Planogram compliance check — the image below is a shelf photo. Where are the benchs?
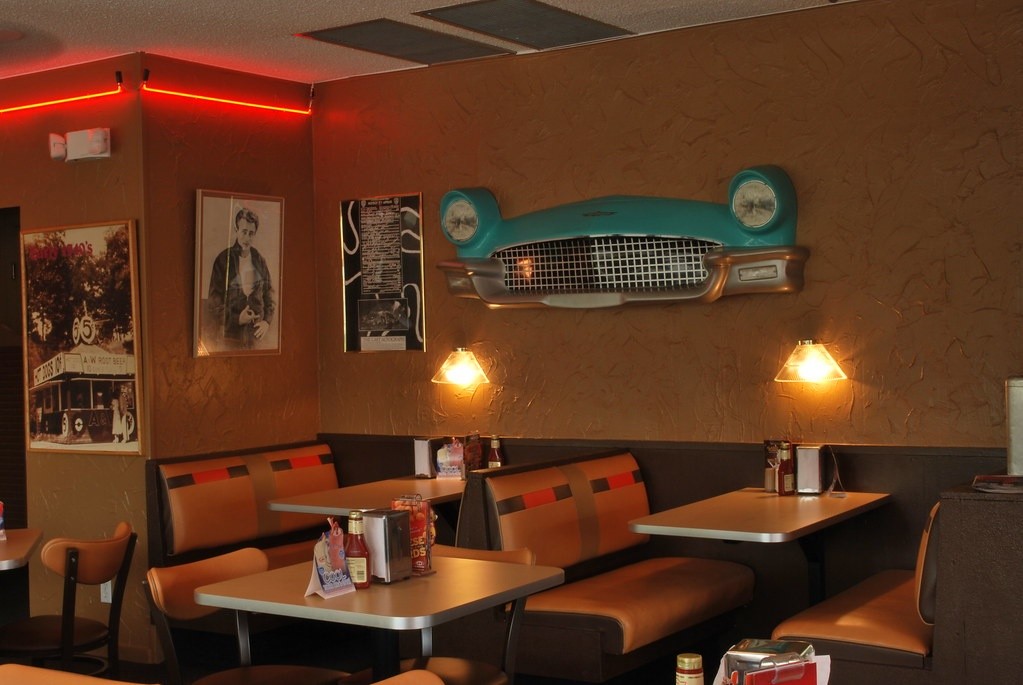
[143,430,947,685]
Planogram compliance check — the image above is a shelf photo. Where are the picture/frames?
[195,189,288,361]
[19,219,145,454]
[339,192,429,355]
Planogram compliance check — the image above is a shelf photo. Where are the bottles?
[675,654,705,685]
[344,511,371,589]
[777,443,795,496]
[488,434,502,468]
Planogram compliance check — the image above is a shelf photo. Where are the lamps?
[771,341,846,384]
[432,349,490,388]
[49,124,112,165]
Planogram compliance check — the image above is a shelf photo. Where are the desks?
[1,527,40,569]
[272,476,468,546]
[628,485,881,621]
[195,555,564,685]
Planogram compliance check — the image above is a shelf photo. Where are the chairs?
[0,520,510,685]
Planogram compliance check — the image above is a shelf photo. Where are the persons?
[209,208,276,350]
[112,388,127,444]
